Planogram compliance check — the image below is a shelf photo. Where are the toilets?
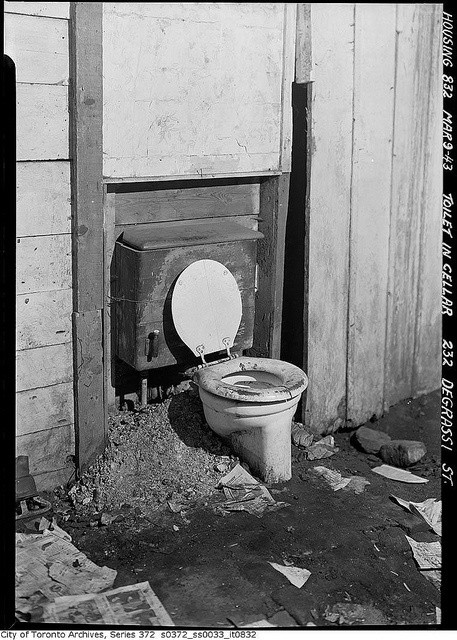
[169,259,307,484]
